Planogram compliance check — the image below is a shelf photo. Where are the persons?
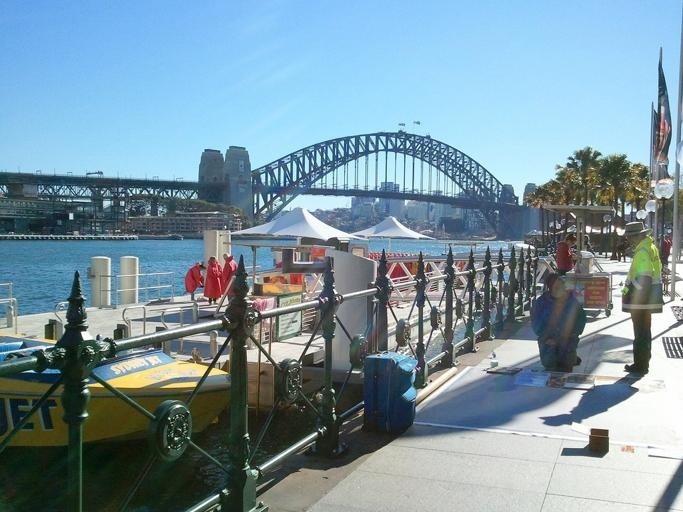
[530,270,587,372]
[556,233,577,276]
[184,262,205,302]
[221,253,238,306]
[204,256,222,306]
[664,234,672,266]
[618,220,664,374]
[657,233,663,249]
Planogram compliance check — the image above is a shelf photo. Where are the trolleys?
[562,272,613,317]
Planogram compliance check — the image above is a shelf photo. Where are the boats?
[1,334,231,447]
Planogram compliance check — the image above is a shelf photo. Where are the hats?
[542,271,558,287]
[622,222,652,238]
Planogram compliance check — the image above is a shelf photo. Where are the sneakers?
[625,364,636,371]
[630,366,649,374]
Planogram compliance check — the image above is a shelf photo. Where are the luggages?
[360,299,418,433]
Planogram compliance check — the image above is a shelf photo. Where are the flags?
[652,61,672,180]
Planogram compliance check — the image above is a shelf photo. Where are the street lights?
[603,213,611,257]
[637,199,657,230]
[653,182,673,258]
